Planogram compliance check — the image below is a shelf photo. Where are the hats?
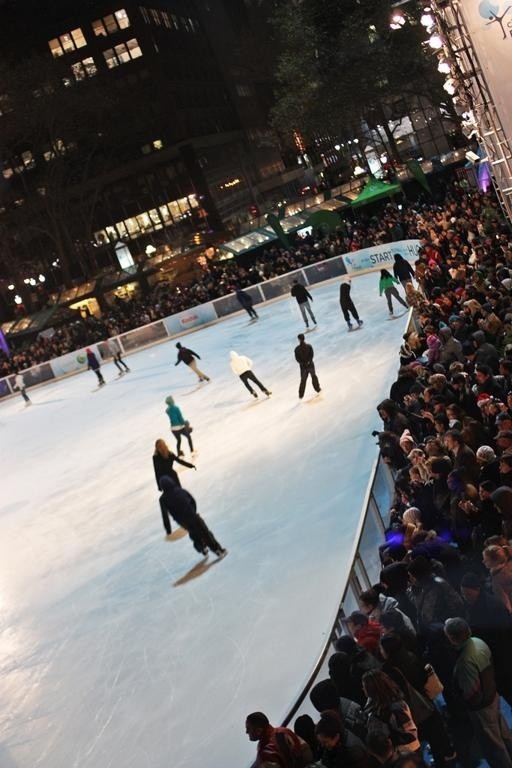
[377,257,512,657]
[341,611,370,629]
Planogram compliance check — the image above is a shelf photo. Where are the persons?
[152,473,230,565]
[244,710,317,767]
[165,394,196,459]
[151,440,197,489]
[236,290,264,320]
[0,274,163,396]
[229,349,273,398]
[173,341,210,382]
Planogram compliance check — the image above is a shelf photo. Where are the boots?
[201,542,228,560]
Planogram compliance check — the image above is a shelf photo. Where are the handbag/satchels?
[388,666,433,722]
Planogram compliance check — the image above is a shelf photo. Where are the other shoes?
[426,742,455,763]
